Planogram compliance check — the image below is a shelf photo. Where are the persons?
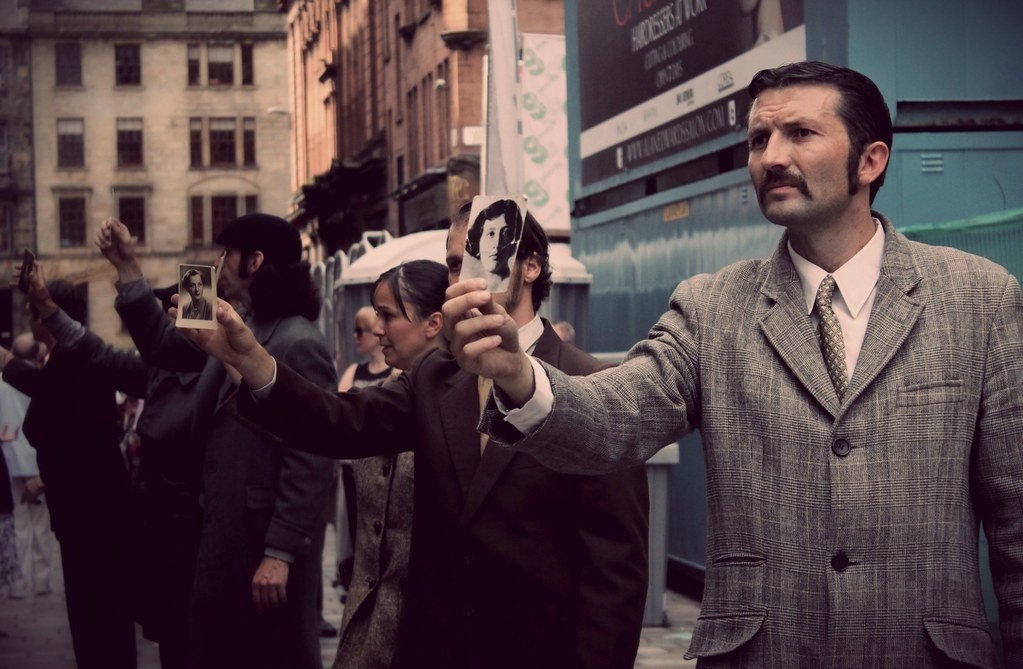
[0,196,650,669]
[181,270,213,321]
[338,306,403,606]
[464,199,524,295]
[439,61,1023,669]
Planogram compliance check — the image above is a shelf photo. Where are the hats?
[10,331,48,363]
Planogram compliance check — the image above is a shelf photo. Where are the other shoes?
[318,617,338,638]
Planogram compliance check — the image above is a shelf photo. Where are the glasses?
[353,325,370,338]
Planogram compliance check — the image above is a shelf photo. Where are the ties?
[813,276,850,404]
[477,376,491,457]
